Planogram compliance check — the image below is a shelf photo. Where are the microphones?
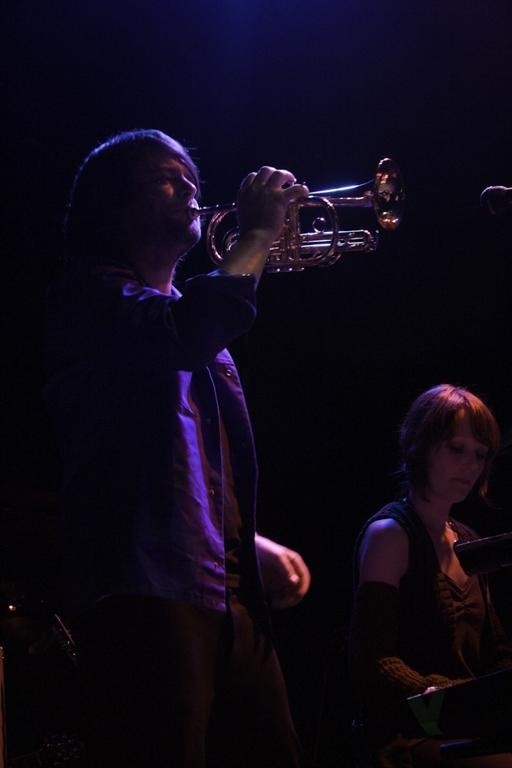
[479,184,512,216]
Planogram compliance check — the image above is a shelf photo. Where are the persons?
[344,383,512,767]
[44,127,313,767]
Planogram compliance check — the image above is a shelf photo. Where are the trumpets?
[194,160,402,276]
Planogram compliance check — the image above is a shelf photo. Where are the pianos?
[407,671,512,744]
